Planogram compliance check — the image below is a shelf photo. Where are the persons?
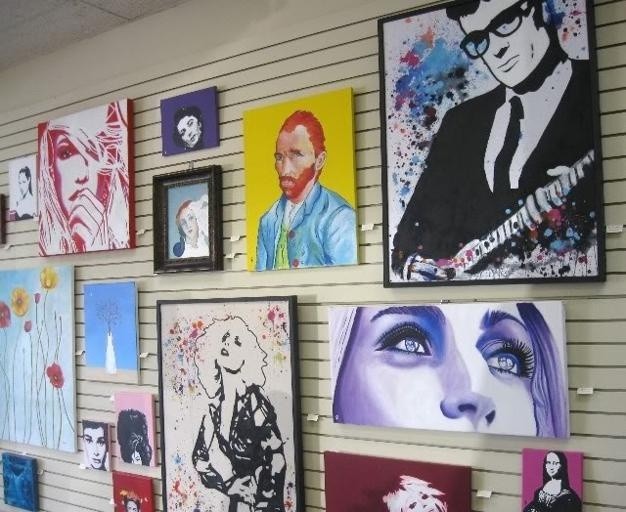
[382,476,448,512]
[14,166,35,220]
[172,106,204,152]
[521,450,582,512]
[327,301,570,439]
[82,419,109,471]
[256,110,358,272]
[116,409,152,467]
[38,99,130,257]
[119,490,142,512]
[191,315,287,512]
[173,201,209,258]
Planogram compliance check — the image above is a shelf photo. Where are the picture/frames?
[152,293,307,512]
[149,163,224,273]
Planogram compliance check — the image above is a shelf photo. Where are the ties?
[274,223,291,270]
[273,149,305,160]
[493,95,524,192]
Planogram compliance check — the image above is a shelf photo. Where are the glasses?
[459,0,536,59]
